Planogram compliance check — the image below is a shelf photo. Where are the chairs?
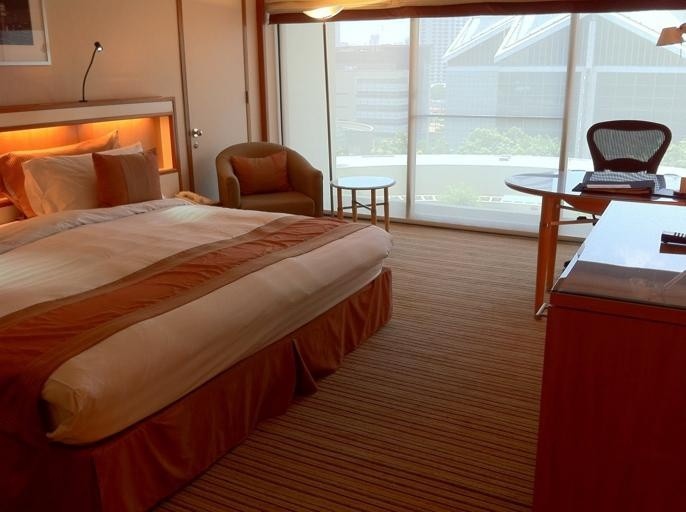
[215,142,323,216]
[585,119,672,174]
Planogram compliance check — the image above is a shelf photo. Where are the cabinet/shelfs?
[530,199,686,512]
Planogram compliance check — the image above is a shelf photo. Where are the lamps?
[79,41,103,102]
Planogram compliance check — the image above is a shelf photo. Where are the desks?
[504,168,686,319]
[330,174,395,232]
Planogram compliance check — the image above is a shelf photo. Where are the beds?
[0,95,392,511]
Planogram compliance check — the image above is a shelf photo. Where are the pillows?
[231,150,291,195]
[20,141,143,217]
[0,129,120,220]
[91,148,164,208]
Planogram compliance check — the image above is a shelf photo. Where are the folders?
[583,171,655,190]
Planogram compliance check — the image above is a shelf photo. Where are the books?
[582,179,655,195]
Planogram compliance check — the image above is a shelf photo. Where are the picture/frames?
[0,0,51,66]
